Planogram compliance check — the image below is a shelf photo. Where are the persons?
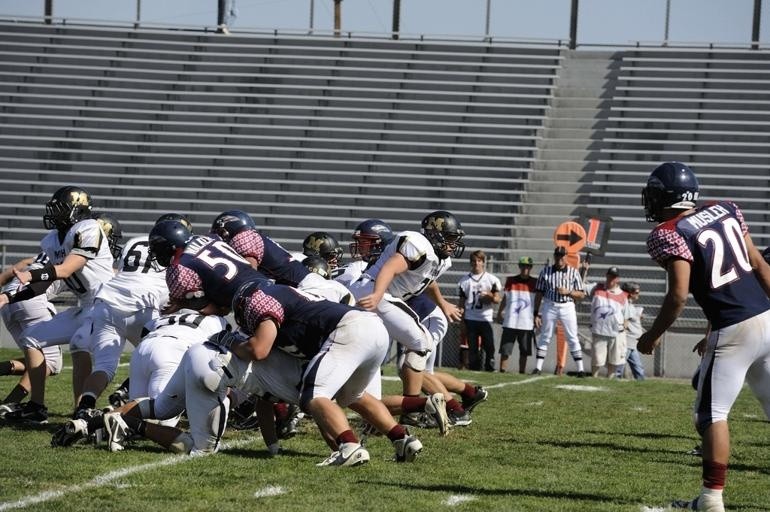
[457,250,501,372]
[613,280,653,382]
[628,159,770,510]
[586,267,628,379]
[2,189,487,467]
[496,255,537,372]
[685,242,769,408]
[530,245,586,379]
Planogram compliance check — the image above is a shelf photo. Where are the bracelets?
[568,289,572,296]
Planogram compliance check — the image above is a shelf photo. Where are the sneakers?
[684,443,702,456]
[0,387,489,468]
[669,486,725,512]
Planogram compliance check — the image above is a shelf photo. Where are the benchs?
[0,21,770,318]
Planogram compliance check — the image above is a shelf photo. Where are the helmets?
[348,219,394,263]
[420,211,465,260]
[301,232,345,265]
[44,187,94,231]
[210,211,256,244]
[96,213,124,261]
[303,255,334,278]
[641,164,700,224]
[154,213,193,235]
[232,280,274,325]
[148,221,194,273]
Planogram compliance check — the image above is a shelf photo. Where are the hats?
[518,256,534,265]
[607,266,618,275]
[553,246,568,257]
